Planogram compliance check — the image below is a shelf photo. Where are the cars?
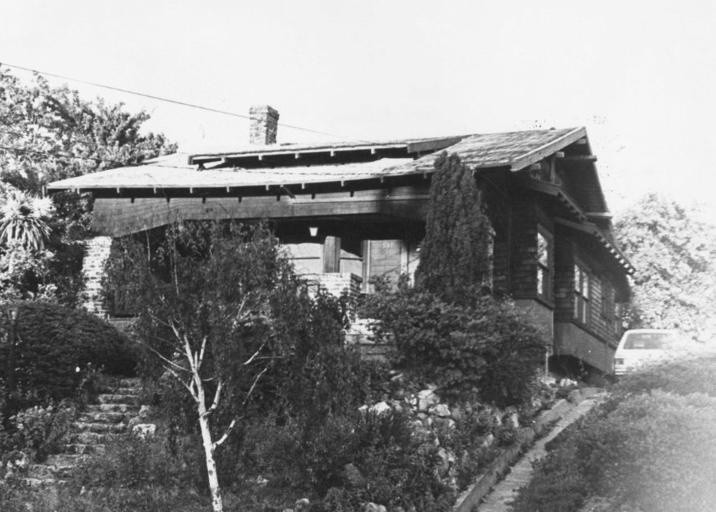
[611,329,686,381]
